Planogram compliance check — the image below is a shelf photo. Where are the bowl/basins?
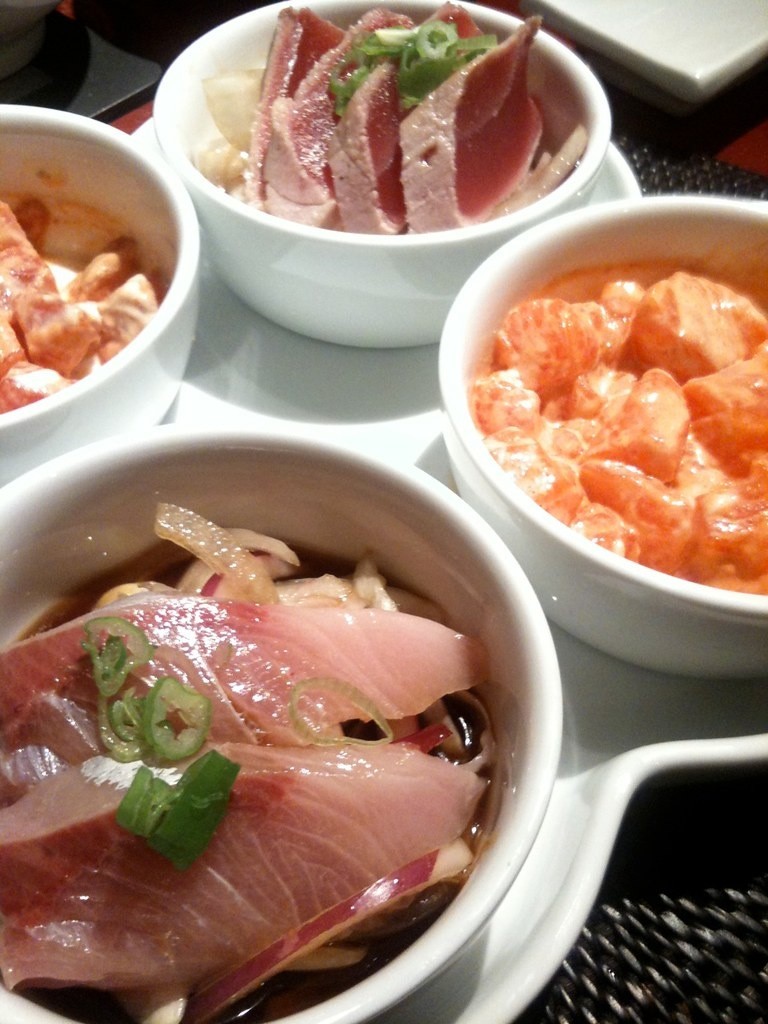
[3,98,205,478]
[442,187,767,685]
[0,1,62,79]
[156,0,612,345]
[0,426,567,1024]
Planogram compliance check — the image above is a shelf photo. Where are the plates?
[5,120,766,1024]
[0,0,252,119]
[520,0,768,114]
